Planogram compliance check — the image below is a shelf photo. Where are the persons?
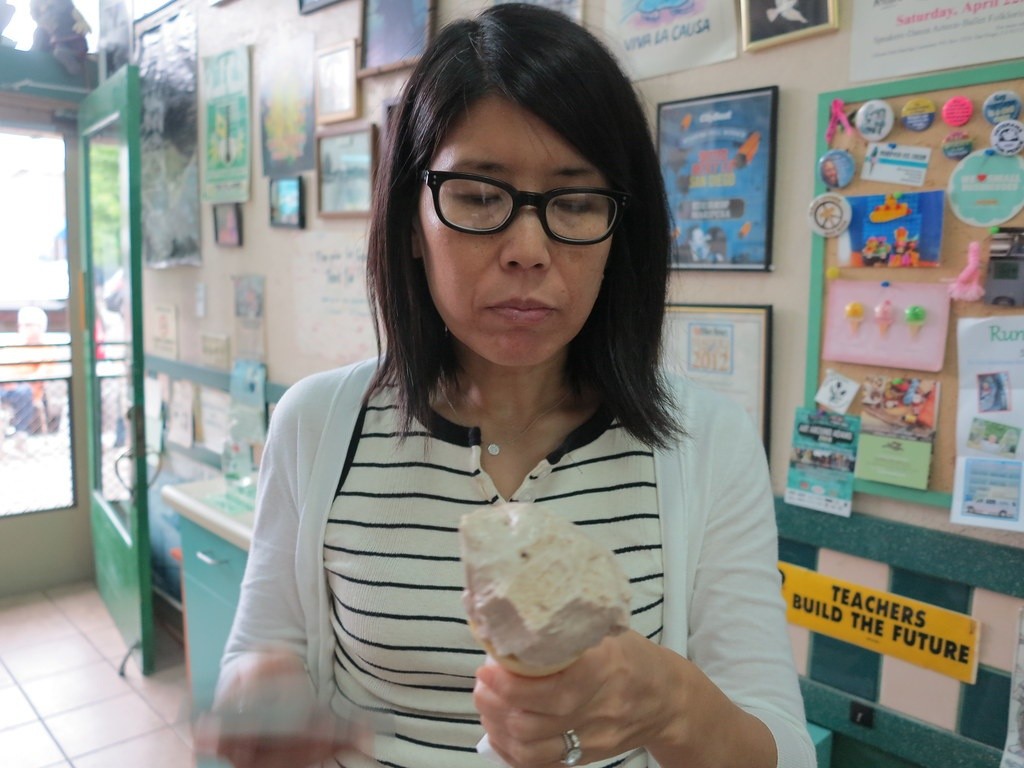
[0,307,56,455]
[193,3,817,768]
[29,0,92,76]
[94,265,132,453]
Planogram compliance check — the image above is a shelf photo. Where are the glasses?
[419,165,626,246]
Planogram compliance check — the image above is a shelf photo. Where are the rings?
[560,729,582,766]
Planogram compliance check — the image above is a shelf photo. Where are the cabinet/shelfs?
[172,518,250,721]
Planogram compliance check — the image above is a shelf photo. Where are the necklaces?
[437,377,573,455]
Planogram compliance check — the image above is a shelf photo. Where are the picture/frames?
[297,0,341,14]
[740,0,839,52]
[213,202,243,246]
[655,86,778,272]
[268,175,306,229]
[315,123,377,218]
[656,301,772,472]
[355,0,436,79]
[314,37,360,124]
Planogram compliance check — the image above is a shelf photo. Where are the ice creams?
[459,502,631,678]
